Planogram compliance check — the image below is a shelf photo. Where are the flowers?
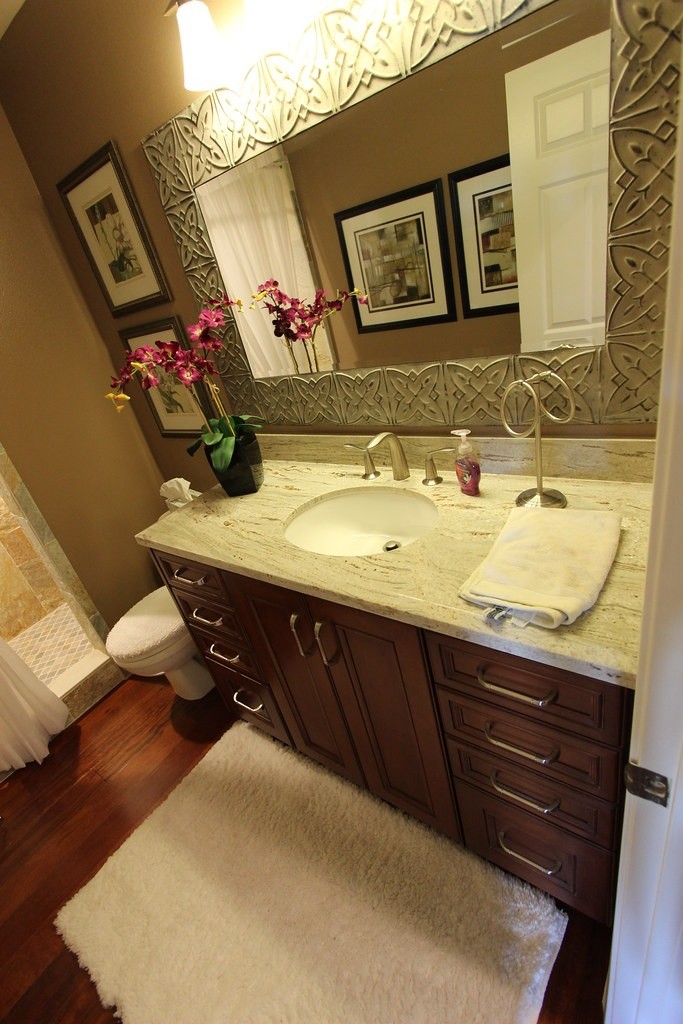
[249,277,369,374]
[104,291,242,413]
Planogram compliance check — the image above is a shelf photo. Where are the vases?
[204,429,264,497]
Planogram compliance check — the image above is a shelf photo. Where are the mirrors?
[140,0,683,427]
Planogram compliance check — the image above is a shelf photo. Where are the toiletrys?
[451,429,480,496]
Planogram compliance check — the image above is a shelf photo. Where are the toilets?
[91,510,216,700]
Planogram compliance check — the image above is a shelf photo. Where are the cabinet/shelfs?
[148,546,635,929]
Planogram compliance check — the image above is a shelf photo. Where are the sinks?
[281,486,439,558]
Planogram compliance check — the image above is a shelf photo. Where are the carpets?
[50,720,568,1024]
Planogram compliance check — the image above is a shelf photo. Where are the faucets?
[366,432,410,481]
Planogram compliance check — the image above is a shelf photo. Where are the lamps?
[162,0,226,92]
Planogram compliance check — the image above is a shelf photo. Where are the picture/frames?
[55,140,174,320]
[117,313,220,439]
[332,177,457,335]
[446,152,521,320]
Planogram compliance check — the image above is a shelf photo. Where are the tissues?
[160,478,203,512]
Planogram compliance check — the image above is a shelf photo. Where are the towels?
[459,508,623,630]
